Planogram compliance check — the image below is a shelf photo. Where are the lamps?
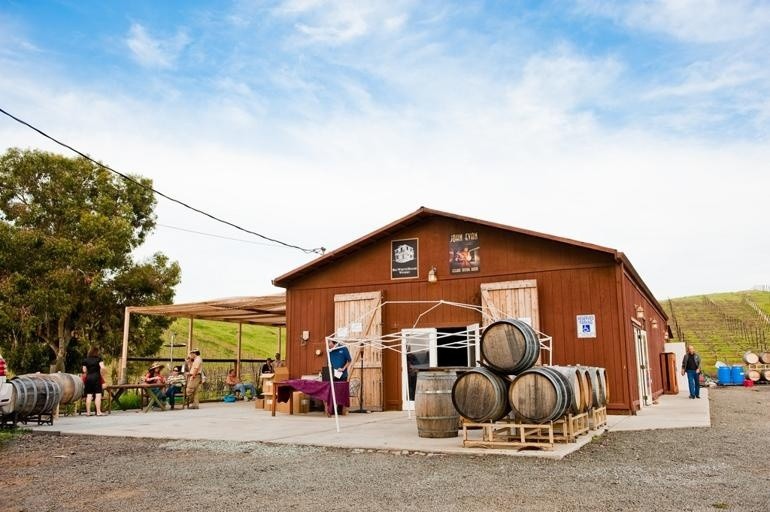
[650,317,657,328]
[634,303,643,318]
[663,330,668,339]
[428,265,437,283]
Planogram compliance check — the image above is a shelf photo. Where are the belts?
[189,373,200,375]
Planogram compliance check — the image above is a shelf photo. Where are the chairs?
[141,377,149,410]
[224,381,247,400]
[79,397,108,414]
[174,376,190,409]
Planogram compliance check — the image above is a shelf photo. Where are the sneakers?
[189,405,199,409]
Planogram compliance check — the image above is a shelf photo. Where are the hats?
[190,348,199,352]
[149,362,163,371]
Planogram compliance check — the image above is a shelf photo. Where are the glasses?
[173,370,179,372]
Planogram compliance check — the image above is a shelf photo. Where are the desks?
[272,381,351,418]
[101,383,166,413]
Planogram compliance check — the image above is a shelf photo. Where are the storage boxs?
[256,367,311,413]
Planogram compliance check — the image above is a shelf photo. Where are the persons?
[326,339,352,416]
[262,357,273,374]
[81,347,108,416]
[144,360,166,411]
[162,364,186,409]
[681,345,701,399]
[184,356,195,374]
[405,344,423,399]
[227,368,257,402]
[270,352,284,373]
[184,347,203,409]
[81,371,107,414]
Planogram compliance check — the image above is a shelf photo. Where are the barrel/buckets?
[452,368,511,421]
[504,367,571,421]
[414,370,458,439]
[2,372,87,418]
[558,364,613,416]
[480,320,540,370]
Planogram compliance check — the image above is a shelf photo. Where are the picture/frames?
[390,238,419,280]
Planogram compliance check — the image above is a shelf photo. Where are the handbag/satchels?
[202,372,206,383]
